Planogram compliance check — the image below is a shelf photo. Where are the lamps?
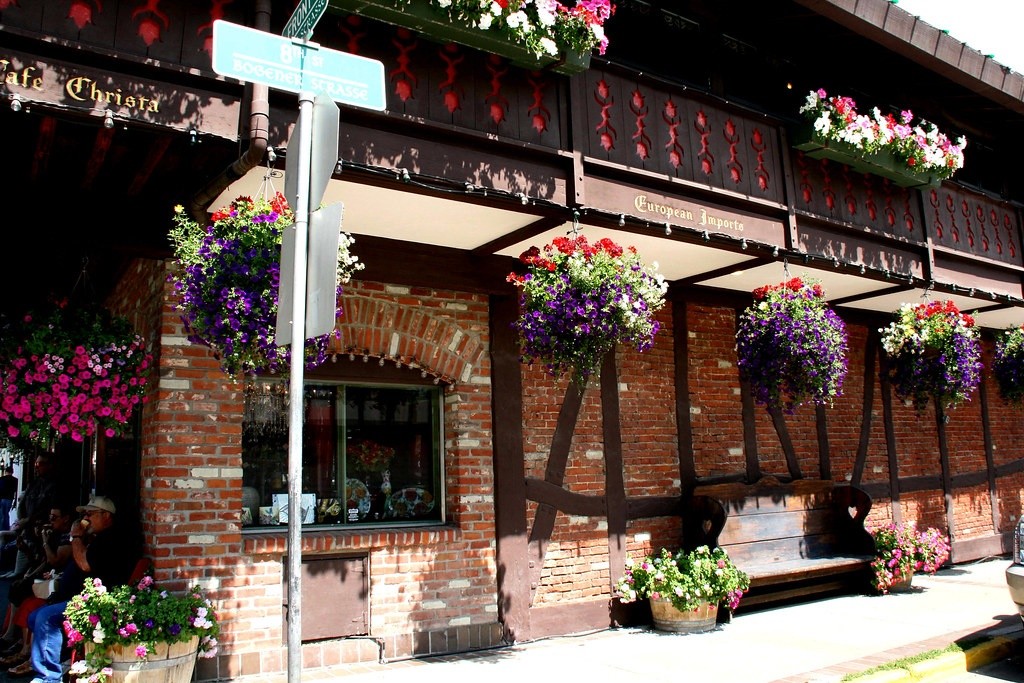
[242,376,307,443]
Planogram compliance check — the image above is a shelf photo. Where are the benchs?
[680,475,878,624]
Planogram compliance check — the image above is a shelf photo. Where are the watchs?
[70,534,82,542]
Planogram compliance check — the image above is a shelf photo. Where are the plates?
[347,478,371,519]
[388,487,435,515]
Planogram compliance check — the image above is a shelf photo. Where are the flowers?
[170,192,365,385]
[800,87,968,182]
[734,273,849,415]
[64,573,221,683]
[878,301,985,423]
[0,297,153,466]
[348,440,395,475]
[391,0,618,60]
[870,520,951,595]
[504,235,669,394]
[612,544,751,613]
[989,323,1024,406]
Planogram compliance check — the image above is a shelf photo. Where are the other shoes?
[0,638,10,648]
[0,653,25,668]
[2,643,23,656]
[6,661,34,678]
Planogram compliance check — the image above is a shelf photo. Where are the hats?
[76,496,116,514]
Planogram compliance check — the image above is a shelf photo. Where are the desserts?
[43,524,52,530]
[81,519,89,528]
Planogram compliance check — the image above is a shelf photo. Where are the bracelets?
[43,542,48,547]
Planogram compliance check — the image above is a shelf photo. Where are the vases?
[85,635,200,683]
[324,0,591,77]
[792,116,942,191]
[363,475,379,510]
[649,592,719,632]
[887,565,914,594]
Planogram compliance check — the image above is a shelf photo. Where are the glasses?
[49,514,61,520]
[82,511,106,517]
[34,462,44,467]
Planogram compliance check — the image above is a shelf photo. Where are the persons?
[0,453,131,676]
[0,467,18,530]
